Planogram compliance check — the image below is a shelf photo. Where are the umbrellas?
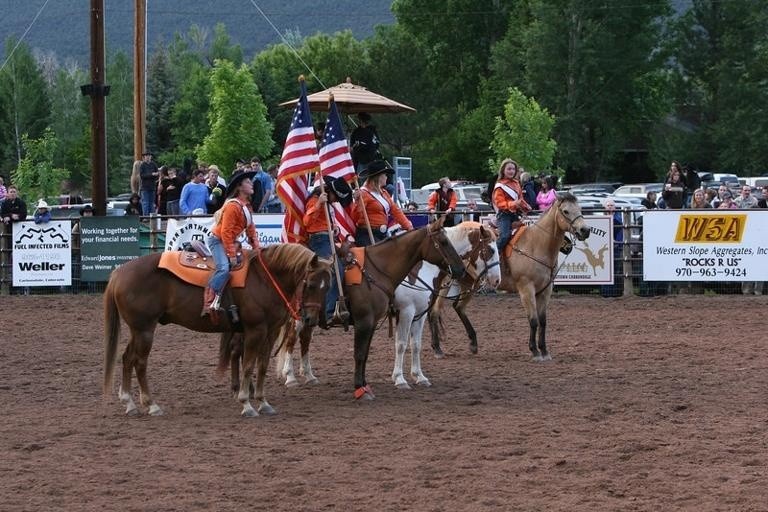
[278,76,419,121]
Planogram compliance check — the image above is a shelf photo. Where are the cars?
[25,192,130,217]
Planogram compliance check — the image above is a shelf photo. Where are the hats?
[358,159,396,180]
[33,199,54,211]
[226,169,257,193]
[487,174,499,199]
[79,205,96,216]
[141,150,155,156]
[322,173,354,208]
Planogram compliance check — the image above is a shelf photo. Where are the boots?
[199,285,226,318]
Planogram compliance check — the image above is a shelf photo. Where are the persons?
[601,161,768,297]
[349,110,380,186]
[201,166,260,325]
[303,176,353,326]
[313,123,325,162]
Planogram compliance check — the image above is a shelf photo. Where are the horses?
[214,213,466,401]
[102,242,340,420]
[275,221,503,390]
[428,188,592,363]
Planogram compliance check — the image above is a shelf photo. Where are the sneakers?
[327,311,351,328]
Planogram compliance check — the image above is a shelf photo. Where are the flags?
[309,92,359,189]
[275,74,322,244]
